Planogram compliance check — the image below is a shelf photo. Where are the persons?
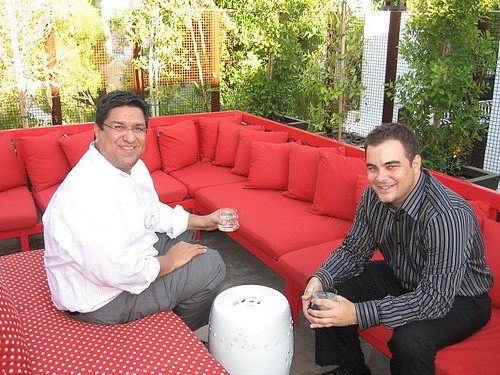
[301,123,495,375]
[41,91,241,332]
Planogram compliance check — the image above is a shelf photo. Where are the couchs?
[0,110,500,375]
[0,244,231,375]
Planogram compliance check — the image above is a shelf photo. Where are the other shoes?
[316,365,372,375]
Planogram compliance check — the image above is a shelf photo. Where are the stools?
[208,284,294,375]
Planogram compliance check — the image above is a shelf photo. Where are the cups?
[217,207,237,232]
[311,285,337,311]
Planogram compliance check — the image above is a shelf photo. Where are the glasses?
[102,123,148,135]
